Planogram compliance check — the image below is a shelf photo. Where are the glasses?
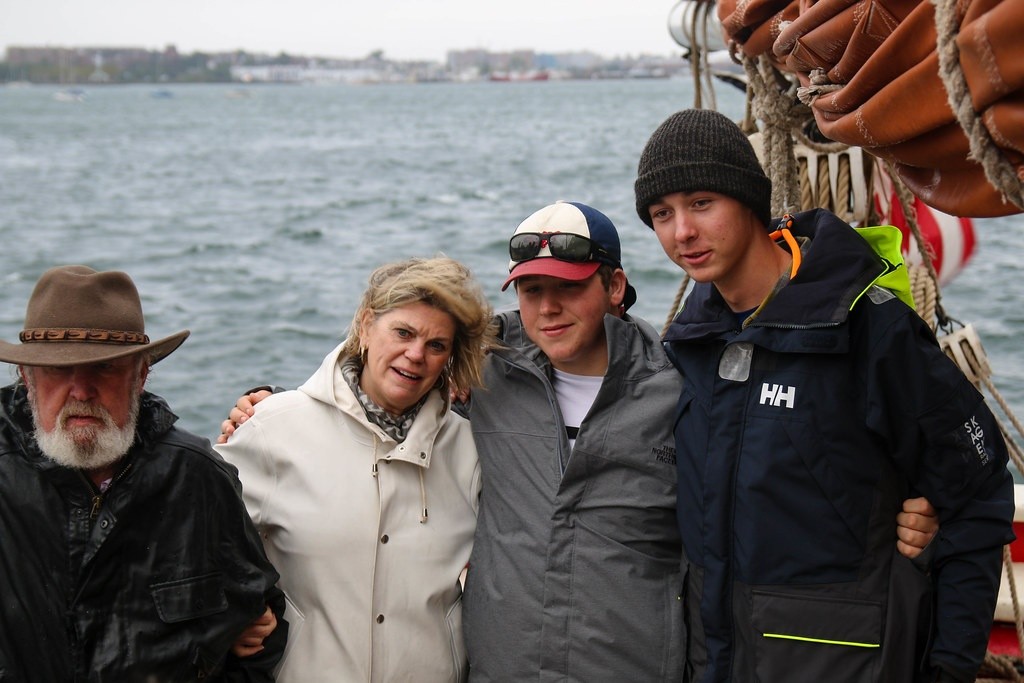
[509,232,621,263]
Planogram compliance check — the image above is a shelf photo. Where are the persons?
[208,255,483,683]
[0,265,286,683]
[220,202,943,683]
[636,109,1015,683]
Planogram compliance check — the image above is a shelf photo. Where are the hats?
[635,109,771,230]
[0,265,191,367]
[501,200,621,292]
